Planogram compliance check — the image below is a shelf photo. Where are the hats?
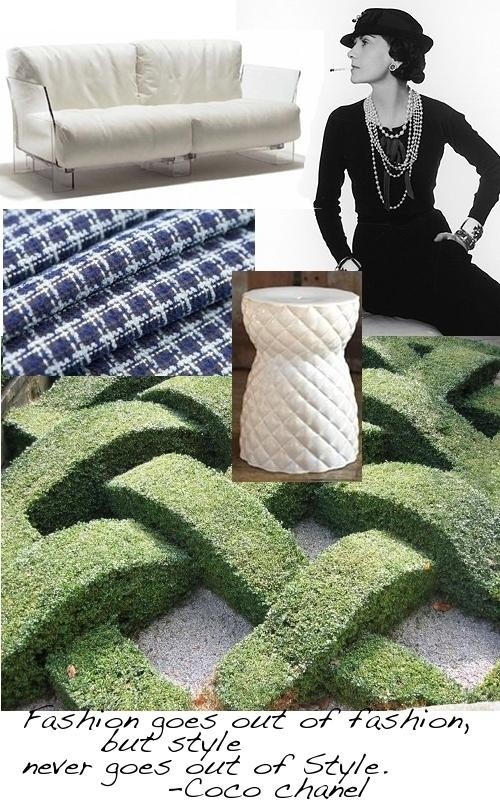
[340,8,433,54]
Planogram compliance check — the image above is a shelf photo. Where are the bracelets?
[456,232,475,250]
[462,215,483,242]
[339,257,363,272]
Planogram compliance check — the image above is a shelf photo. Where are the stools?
[239,286,359,472]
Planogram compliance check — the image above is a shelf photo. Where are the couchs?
[6,40,301,192]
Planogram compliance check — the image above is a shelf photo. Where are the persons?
[312,6,500,336]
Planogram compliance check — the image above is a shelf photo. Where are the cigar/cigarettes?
[330,66,347,72]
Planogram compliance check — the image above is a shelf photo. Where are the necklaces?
[359,87,424,210]
